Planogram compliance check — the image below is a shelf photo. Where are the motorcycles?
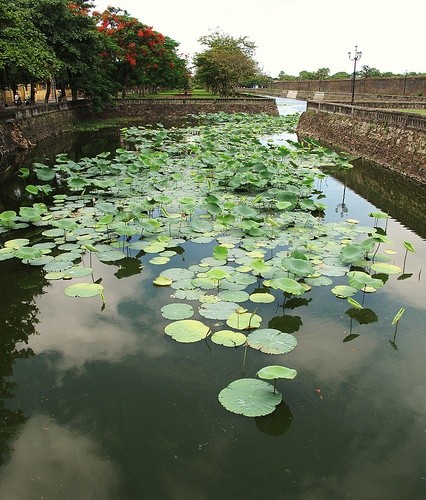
[57,96,64,102]
[14,98,22,107]
[25,100,30,106]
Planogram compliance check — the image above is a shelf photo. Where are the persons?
[11,84,64,109]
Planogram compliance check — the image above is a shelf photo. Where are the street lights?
[348,46,363,106]
